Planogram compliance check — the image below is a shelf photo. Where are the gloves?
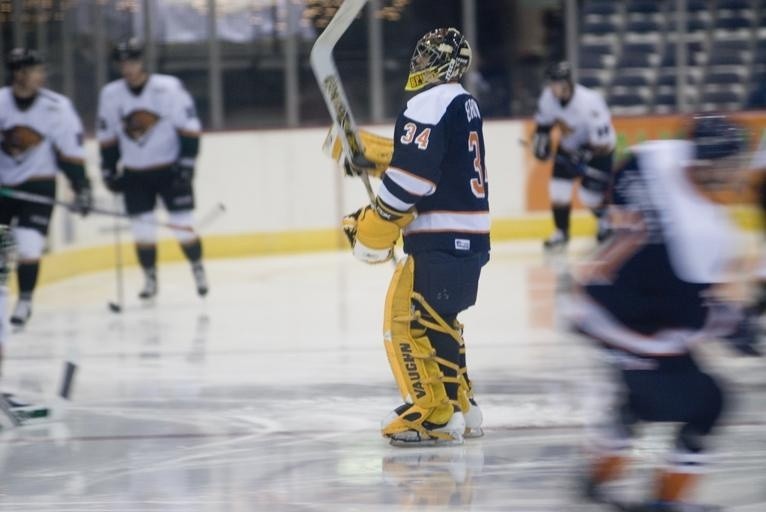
[341,195,417,266]
[71,176,94,217]
[323,120,395,179]
[166,156,199,192]
[102,161,127,195]
[534,131,554,162]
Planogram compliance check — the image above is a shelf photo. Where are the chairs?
[0,1,766,134]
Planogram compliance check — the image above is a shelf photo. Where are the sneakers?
[543,227,572,249]
[191,263,210,296]
[380,401,484,437]
[11,298,34,326]
[138,275,159,301]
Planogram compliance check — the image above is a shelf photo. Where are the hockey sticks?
[109,193,121,313]
[309,1,401,269]
[0,188,227,231]
[5,353,79,428]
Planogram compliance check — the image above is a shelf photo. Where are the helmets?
[403,27,473,92]
[5,46,46,77]
[545,61,574,83]
[112,36,146,63]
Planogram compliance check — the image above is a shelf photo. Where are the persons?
[322,27,489,448]
[565,113,747,510]
[95,39,210,301]
[726,135,766,360]
[532,61,616,246]
[0,47,92,329]
